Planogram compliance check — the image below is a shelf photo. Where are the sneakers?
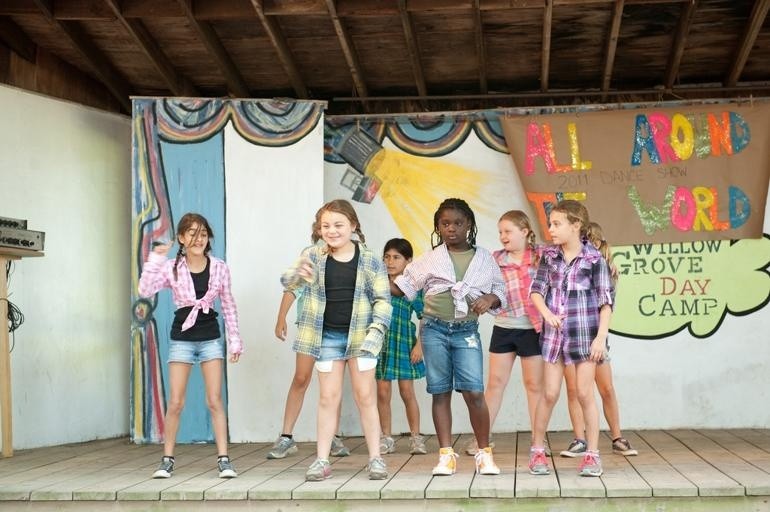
[152,458,175,478]
[579,454,603,476]
[368,459,388,480]
[380,436,395,455]
[475,448,500,475]
[306,460,333,481]
[543,441,551,456]
[559,439,586,458]
[409,431,427,454]
[466,437,495,455]
[267,437,297,459]
[331,437,351,456]
[612,438,638,456]
[528,451,550,475]
[433,447,459,476]
[218,457,237,478]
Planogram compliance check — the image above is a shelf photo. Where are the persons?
[528,199,616,478]
[266,205,351,462]
[271,198,392,482]
[562,222,640,457]
[376,239,434,455]
[135,213,242,480]
[376,198,508,474]
[468,211,554,459]
